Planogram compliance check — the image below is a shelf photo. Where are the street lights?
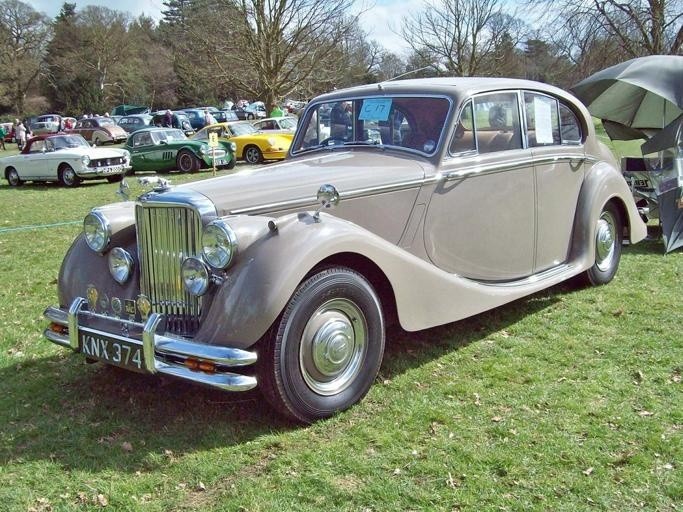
[384,61,448,80]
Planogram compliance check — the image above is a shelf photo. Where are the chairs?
[449,130,561,156]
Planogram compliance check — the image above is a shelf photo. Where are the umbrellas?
[567,54,682,167]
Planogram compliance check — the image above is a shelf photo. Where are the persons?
[489,100,508,128]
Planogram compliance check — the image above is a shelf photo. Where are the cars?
[42,75,648,432]
